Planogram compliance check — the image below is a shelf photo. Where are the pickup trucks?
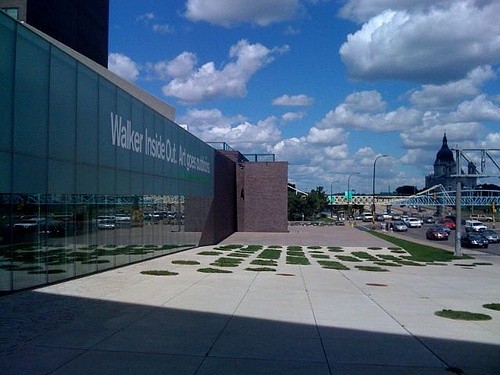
[469,214,493,222]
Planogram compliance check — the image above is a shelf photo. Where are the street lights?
[347,172,361,219]
[373,154,390,224]
[331,181,340,218]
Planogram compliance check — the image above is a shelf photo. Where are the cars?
[433,211,452,217]
[462,231,488,249]
[356,205,435,225]
[445,215,465,225]
[435,223,450,234]
[407,217,421,227]
[442,218,456,230]
[426,227,448,242]
[465,220,487,232]
[480,229,499,243]
[393,220,408,233]
